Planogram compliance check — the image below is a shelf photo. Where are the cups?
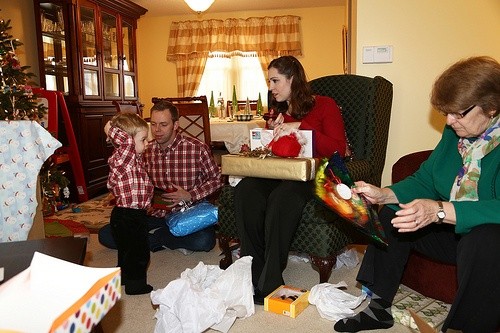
[45,19,125,40]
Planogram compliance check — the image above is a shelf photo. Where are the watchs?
[436,201,445,224]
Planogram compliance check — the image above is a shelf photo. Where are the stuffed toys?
[267,130,306,158]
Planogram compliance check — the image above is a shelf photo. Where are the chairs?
[151,75,458,304]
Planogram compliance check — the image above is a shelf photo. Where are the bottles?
[209,85,262,119]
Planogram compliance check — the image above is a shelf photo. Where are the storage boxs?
[264,285,310,319]
[221,129,322,182]
[0,251,120,333]
[391,288,452,331]
[167,202,220,236]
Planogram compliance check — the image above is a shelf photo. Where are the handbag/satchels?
[165,200,219,236]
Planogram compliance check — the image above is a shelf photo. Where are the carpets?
[59,191,115,233]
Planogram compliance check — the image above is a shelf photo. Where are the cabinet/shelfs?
[34,0,148,201]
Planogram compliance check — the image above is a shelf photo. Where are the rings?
[280,128,282,130]
[414,220,418,226]
[278,123,280,125]
[283,130,285,131]
[171,199,173,202]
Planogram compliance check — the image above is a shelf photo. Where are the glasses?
[442,104,477,119]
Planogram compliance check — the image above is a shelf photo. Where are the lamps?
[184,0,216,14]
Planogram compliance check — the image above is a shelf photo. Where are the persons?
[105,111,155,295]
[98,100,222,256]
[234,55,346,305]
[334,55,500,333]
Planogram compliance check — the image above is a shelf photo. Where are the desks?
[0,234,88,285]
[0,119,63,241]
[210,117,268,153]
